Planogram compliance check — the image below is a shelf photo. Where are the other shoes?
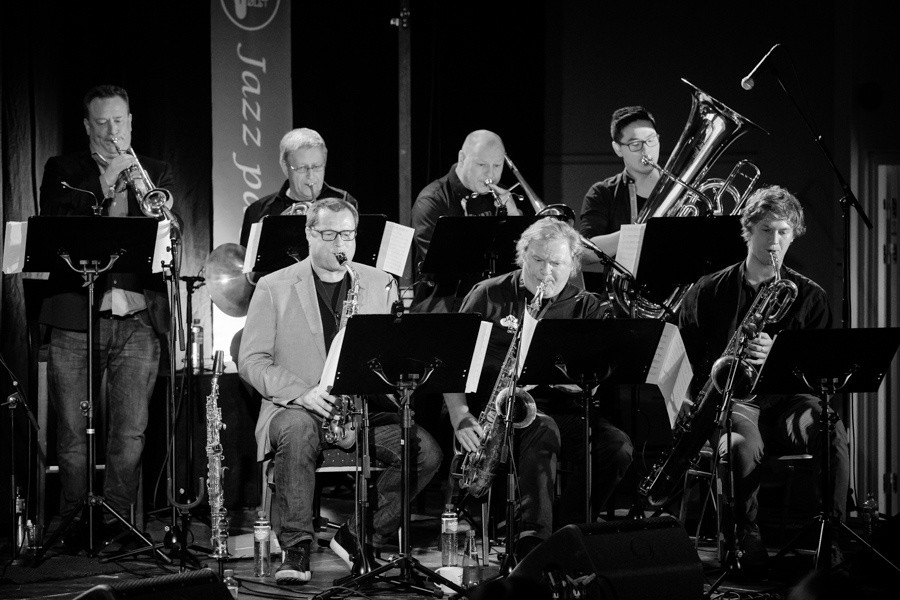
[813,545,849,578]
[519,524,550,542]
[103,518,153,546]
[44,534,71,547]
[735,523,766,564]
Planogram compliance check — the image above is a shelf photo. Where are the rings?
[761,346,764,353]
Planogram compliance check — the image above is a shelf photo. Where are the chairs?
[262,447,403,558]
[679,448,814,532]
[437,427,492,567]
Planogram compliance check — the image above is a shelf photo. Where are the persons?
[231,101,853,589]
[35,85,186,560]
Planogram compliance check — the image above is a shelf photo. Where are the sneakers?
[329,520,386,578]
[275,546,312,584]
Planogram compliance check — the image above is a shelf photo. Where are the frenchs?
[202,183,315,318]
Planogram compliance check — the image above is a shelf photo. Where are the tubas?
[604,76,772,322]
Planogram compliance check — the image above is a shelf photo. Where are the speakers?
[73,566,234,600]
[494,516,704,600]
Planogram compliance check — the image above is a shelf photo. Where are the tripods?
[313,313,482,600]
[702,328,900,600]
[22,214,213,574]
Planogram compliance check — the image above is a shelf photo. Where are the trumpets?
[504,153,576,230]
[112,137,175,218]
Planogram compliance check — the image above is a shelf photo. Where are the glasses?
[615,134,659,152]
[310,226,357,241]
[285,158,326,173]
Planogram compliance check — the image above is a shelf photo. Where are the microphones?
[159,206,181,231]
[740,44,780,90]
[740,323,758,336]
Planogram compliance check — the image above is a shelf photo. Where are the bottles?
[253,511,271,577]
[191,319,203,375]
[462,531,481,589]
[440,504,459,567]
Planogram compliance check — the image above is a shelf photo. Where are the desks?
[149,371,262,512]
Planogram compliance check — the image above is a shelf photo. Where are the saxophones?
[637,251,798,507]
[319,252,361,451]
[202,349,230,557]
[450,279,547,498]
